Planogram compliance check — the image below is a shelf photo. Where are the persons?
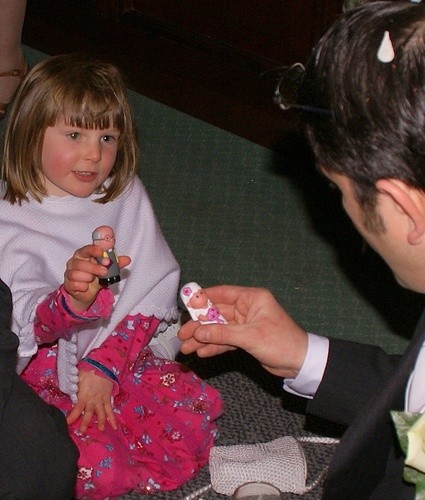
[92,225,121,285]
[0,0,33,120]
[0,51,226,499]
[177,0,425,500]
[180,281,228,327]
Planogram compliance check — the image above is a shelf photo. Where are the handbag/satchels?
[208,435,342,496]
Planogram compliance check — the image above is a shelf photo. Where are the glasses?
[272,62,332,121]
